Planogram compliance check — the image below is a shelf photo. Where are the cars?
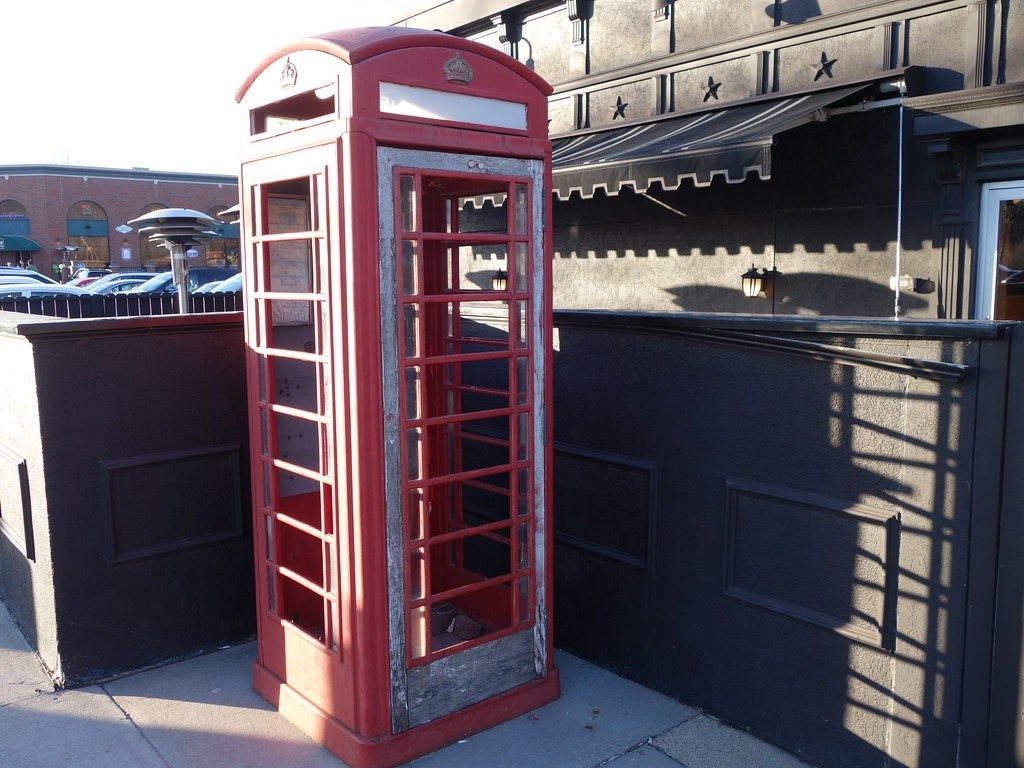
[68,268,113,280]
[0,264,104,299]
[63,273,161,296]
[191,271,244,293]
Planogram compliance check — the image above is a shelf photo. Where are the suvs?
[115,265,241,296]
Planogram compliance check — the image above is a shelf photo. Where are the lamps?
[493,268,508,290]
[740,263,769,299]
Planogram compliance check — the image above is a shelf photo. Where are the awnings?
[0,235,42,251]
[459,83,877,211]
